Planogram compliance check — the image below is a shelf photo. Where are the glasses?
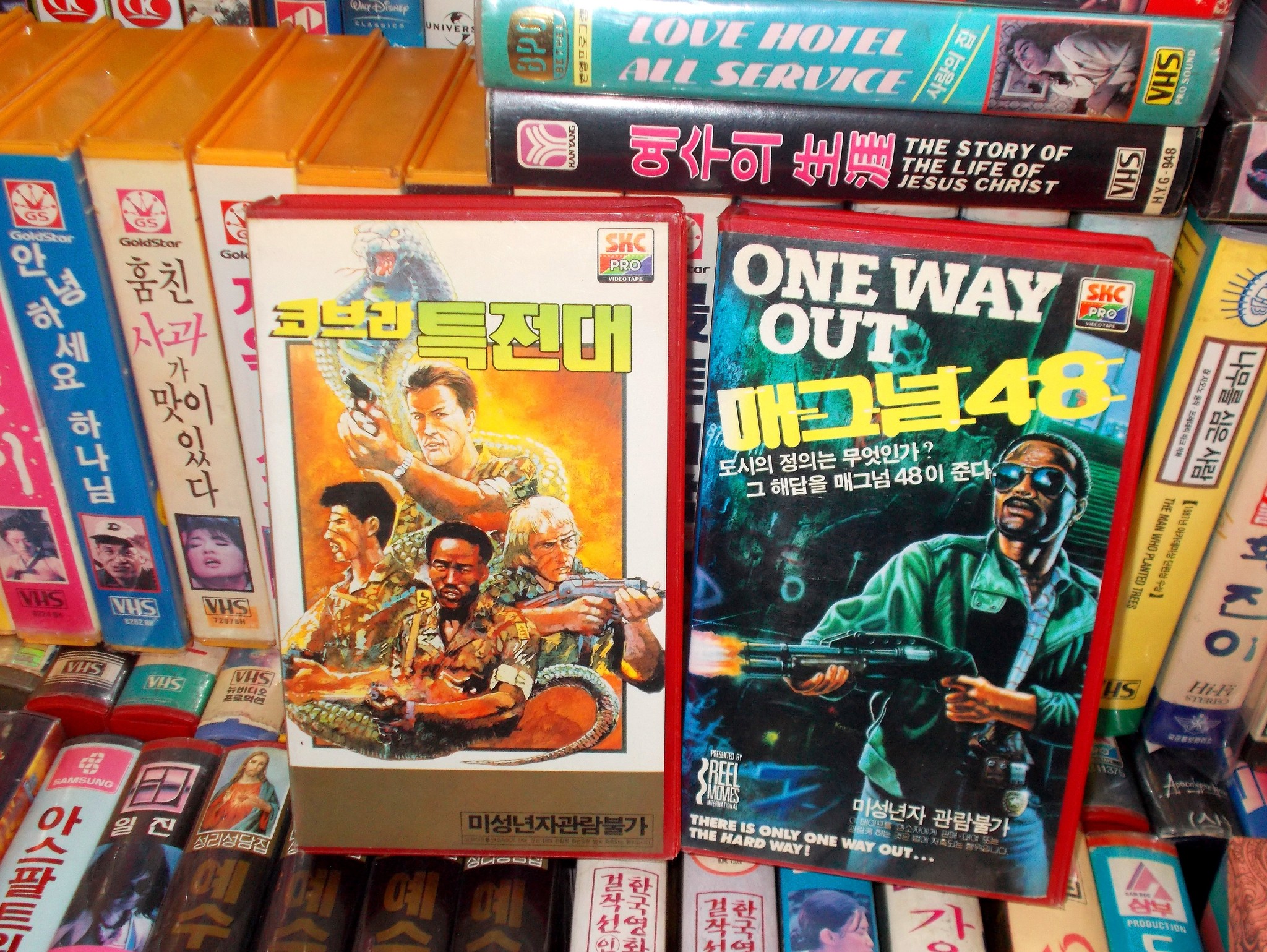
[992,462,1079,498]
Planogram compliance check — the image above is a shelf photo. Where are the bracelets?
[392,451,414,480]
[405,700,416,719]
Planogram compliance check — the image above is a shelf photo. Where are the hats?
[89,520,140,545]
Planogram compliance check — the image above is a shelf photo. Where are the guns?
[737,631,980,735]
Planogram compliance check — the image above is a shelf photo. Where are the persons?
[789,888,876,952]
[199,751,280,837]
[0,515,66,581]
[784,427,1102,900]
[88,519,155,590]
[1007,24,1142,123]
[181,519,252,590]
[46,842,171,950]
[284,362,666,734]
